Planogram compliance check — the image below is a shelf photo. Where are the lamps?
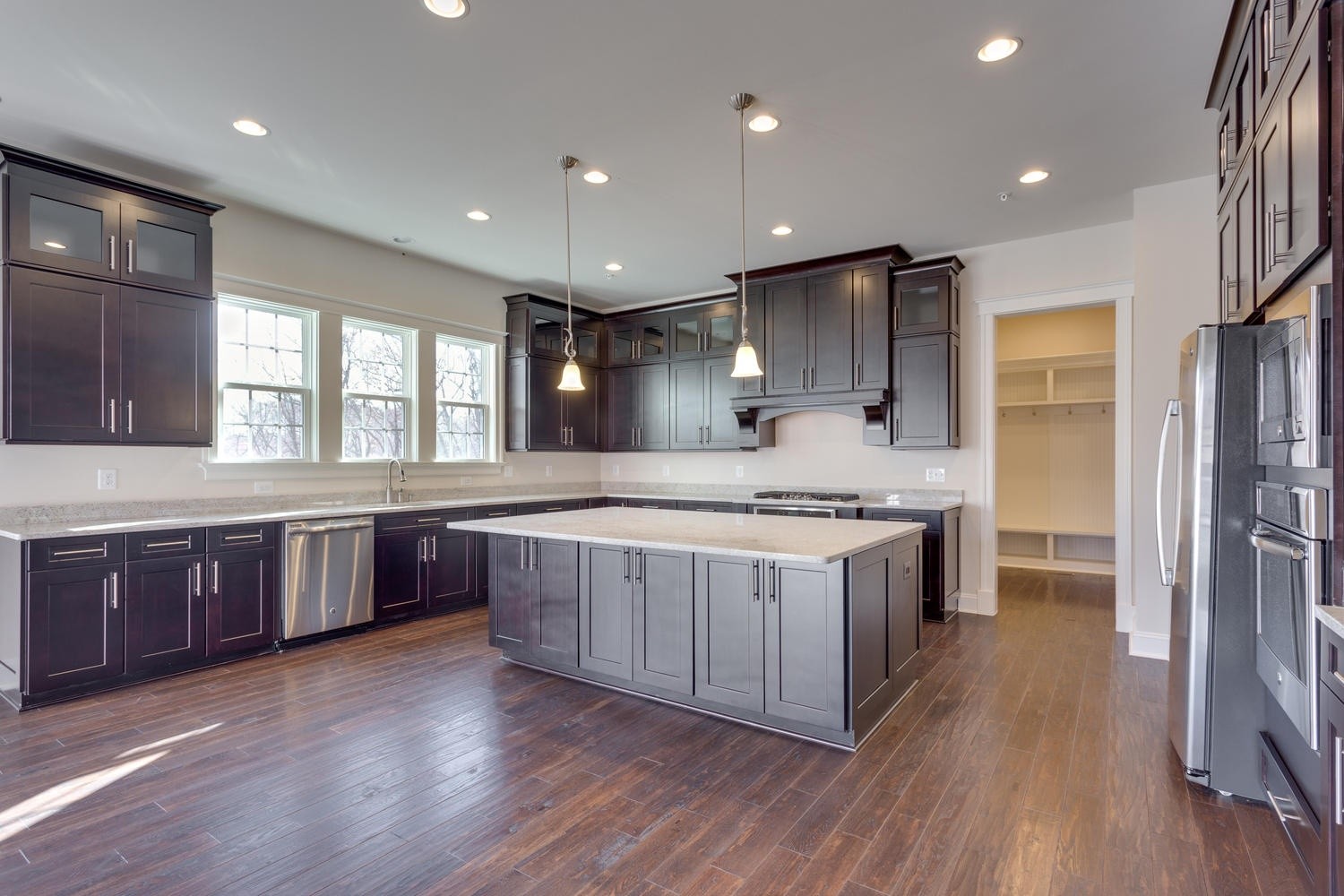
[555,155,586,391]
[728,93,764,378]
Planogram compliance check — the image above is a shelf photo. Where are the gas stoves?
[754,491,860,502]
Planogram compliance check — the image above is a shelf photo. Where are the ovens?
[753,506,836,519]
[1248,481,1328,750]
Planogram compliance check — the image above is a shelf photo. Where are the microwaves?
[1259,319,1306,445]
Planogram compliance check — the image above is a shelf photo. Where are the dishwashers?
[282,516,375,642]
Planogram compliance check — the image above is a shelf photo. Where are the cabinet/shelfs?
[994,351,1116,575]
[1204,0,1333,322]
[1258,604,1344,896]
[0,142,226,448]
[0,521,281,713]
[502,244,966,453]
[374,497,963,753]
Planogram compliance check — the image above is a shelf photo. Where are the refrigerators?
[1156,323,1266,802]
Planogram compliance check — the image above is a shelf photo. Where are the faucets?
[386,459,407,503]
